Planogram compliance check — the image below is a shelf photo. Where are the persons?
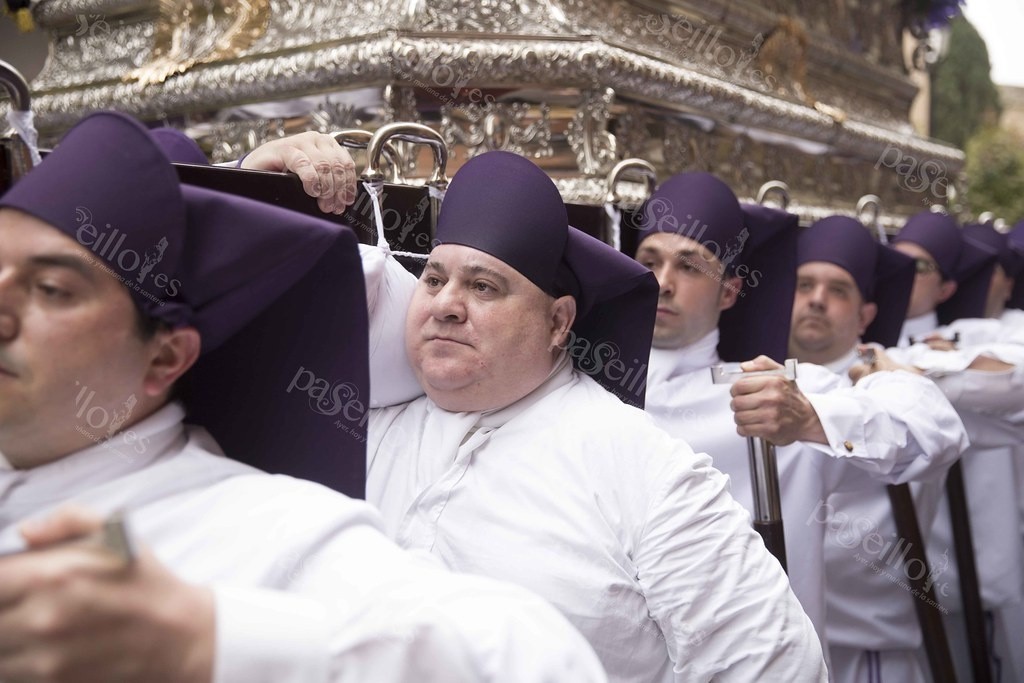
[625,171,971,682]
[785,215,1022,683]
[965,223,1022,333]
[214,129,832,683]
[882,211,1023,683]
[0,107,613,683]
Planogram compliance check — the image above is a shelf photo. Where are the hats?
[893,210,1000,326]
[960,223,1024,312]
[638,170,801,369]
[0,112,368,502]
[802,214,916,349]
[439,148,659,411]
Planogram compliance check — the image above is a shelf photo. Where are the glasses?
[915,256,938,275]
